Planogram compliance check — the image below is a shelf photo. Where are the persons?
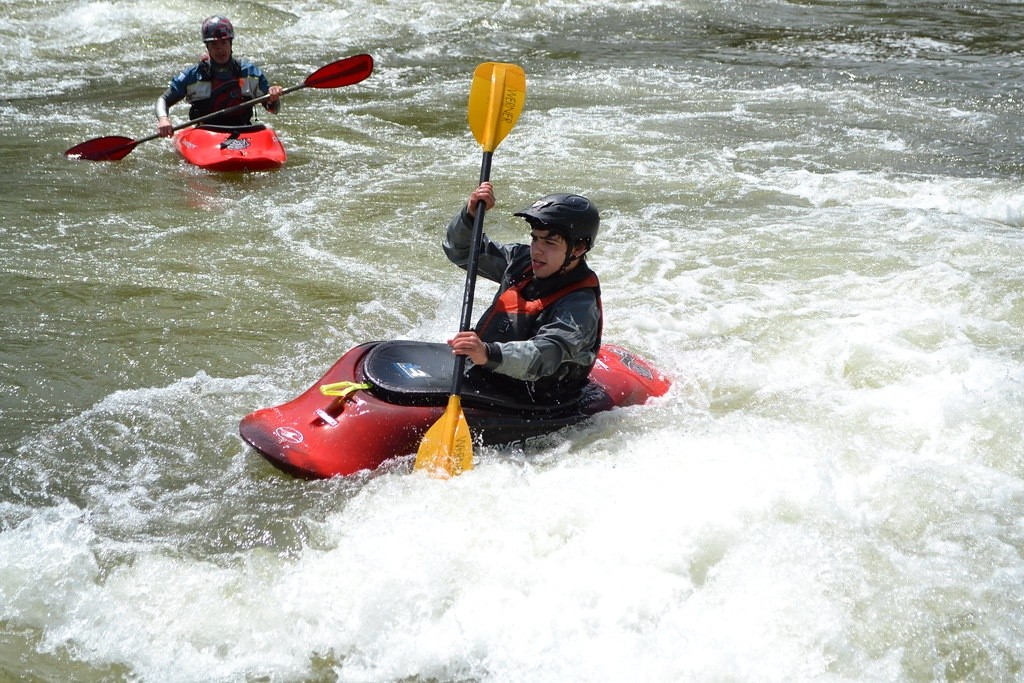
[442,181,605,395]
[154,14,282,136]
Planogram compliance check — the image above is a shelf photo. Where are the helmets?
[514,193,599,246]
[201,15,234,42]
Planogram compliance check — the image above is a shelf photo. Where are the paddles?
[63,54,375,162]
[412,62,527,479]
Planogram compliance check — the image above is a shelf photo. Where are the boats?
[174,123,287,171]
[237,339,677,479]
